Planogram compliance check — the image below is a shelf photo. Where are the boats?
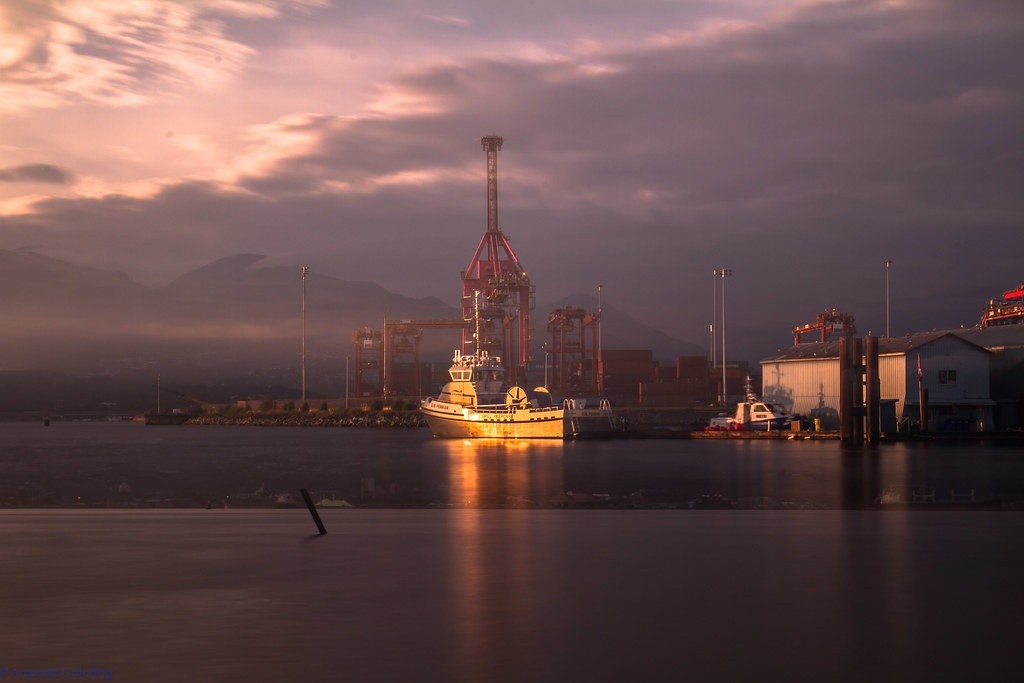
[418,350,632,441]
[144,411,192,426]
[704,391,794,432]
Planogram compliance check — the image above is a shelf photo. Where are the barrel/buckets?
[814,419,824,431]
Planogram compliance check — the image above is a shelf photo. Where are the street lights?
[721,268,732,402]
[597,284,603,395]
[885,258,892,339]
[299,265,309,411]
[712,268,717,370]
